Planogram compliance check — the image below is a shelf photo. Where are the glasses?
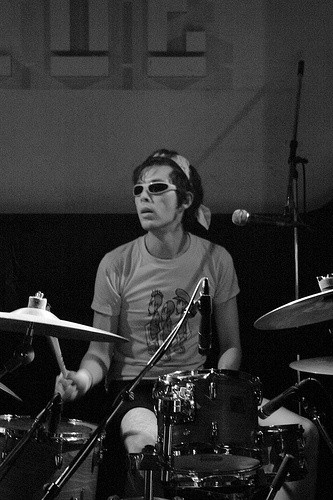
[131,182,185,197]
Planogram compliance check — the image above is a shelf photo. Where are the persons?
[55,149,319,500]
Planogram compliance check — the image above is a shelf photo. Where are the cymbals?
[0,292,128,342]
[252,276,333,329]
[288,355,333,375]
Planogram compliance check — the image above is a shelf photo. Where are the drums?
[256,423,308,483]
[0,413,106,500]
[154,369,263,477]
